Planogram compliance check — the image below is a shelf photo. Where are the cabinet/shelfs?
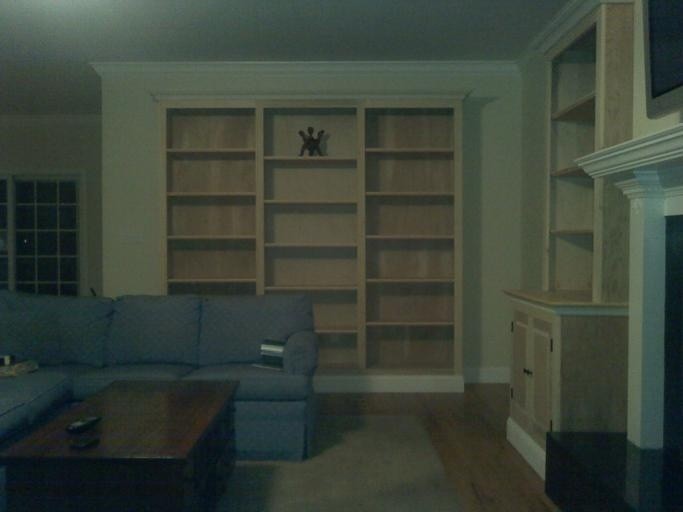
[0,173,82,297]
[540,1,633,304]
[157,97,464,396]
[504,291,628,462]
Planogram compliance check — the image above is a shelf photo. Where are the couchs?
[1,289,320,511]
